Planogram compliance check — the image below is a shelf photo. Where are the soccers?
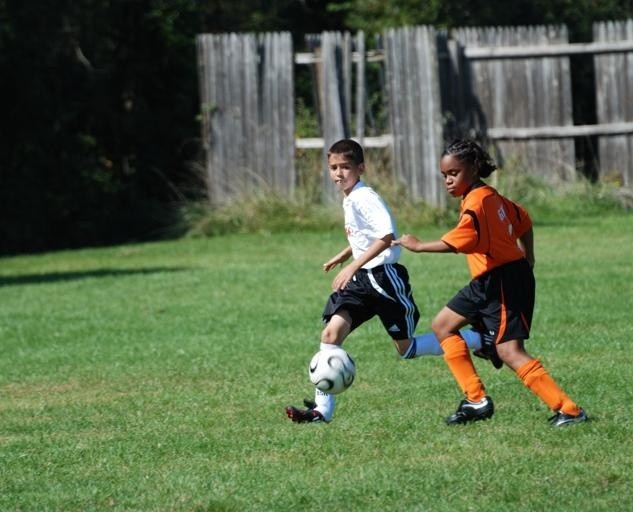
[310,348,355,394]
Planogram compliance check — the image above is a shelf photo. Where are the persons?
[286,140,504,425]
[390,140,587,430]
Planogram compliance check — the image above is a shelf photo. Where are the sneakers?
[547,407,587,426]
[471,326,504,369]
[447,396,494,424]
[285,399,329,423]
